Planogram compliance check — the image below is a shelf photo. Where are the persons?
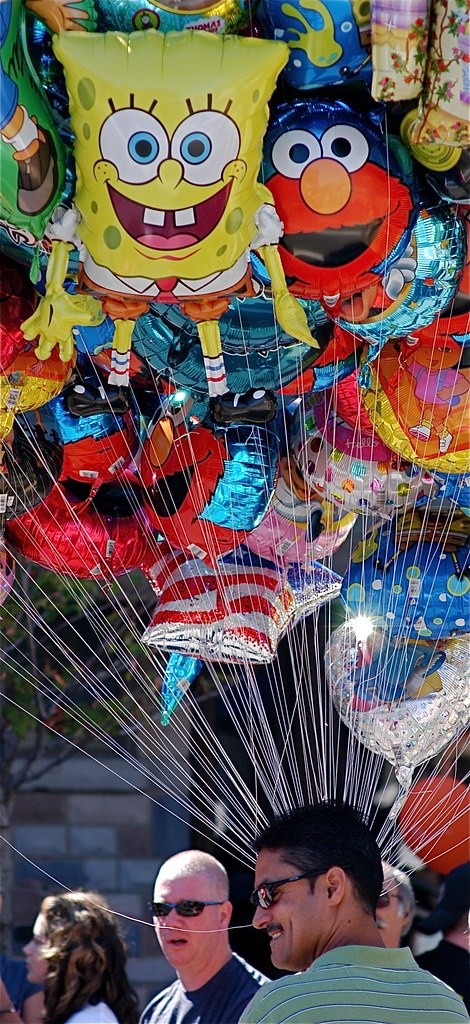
[238,800,470,1024]
[0,891,141,1024]
[138,850,273,1024]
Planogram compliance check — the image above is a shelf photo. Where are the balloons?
[398,778,470,874]
[0,0,470,793]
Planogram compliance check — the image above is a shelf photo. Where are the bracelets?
[0,1007,16,1014]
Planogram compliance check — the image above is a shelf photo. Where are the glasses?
[147,900,223,917]
[376,889,400,908]
[250,865,349,910]
